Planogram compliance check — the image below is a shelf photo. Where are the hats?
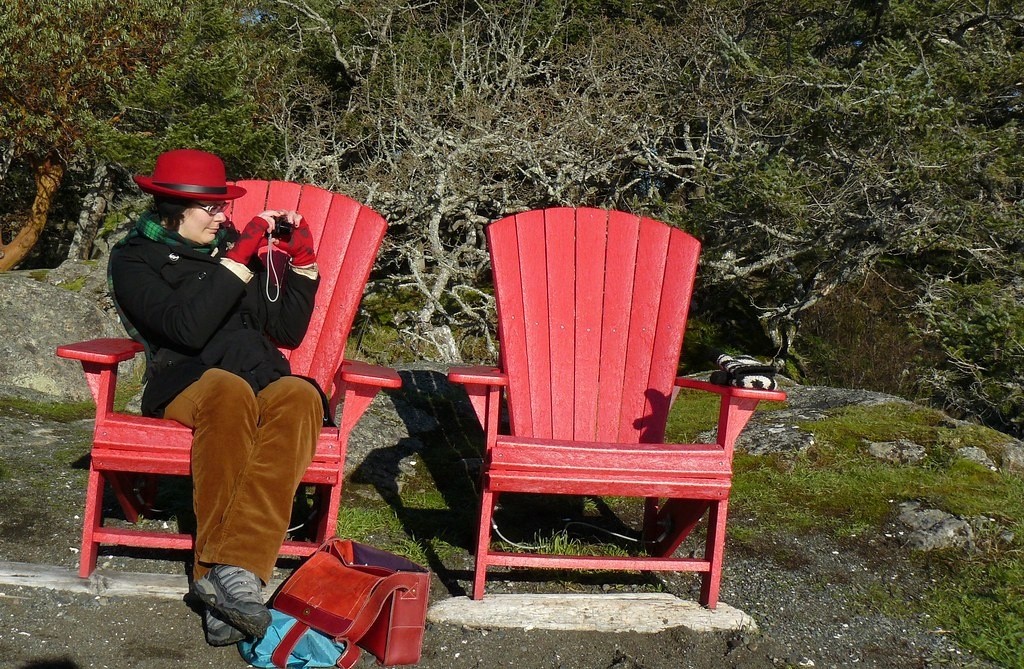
[133,149,247,200]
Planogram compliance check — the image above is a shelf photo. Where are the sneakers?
[206,608,246,646]
[192,564,272,638]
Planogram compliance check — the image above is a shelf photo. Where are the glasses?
[187,199,230,216]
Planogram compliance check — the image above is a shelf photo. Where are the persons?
[106,150,330,648]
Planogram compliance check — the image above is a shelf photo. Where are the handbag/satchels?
[271,537,430,669]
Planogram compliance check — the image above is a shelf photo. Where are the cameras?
[264,216,293,242]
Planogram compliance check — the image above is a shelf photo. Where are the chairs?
[449,208,787,615]
[56,178,406,575]
